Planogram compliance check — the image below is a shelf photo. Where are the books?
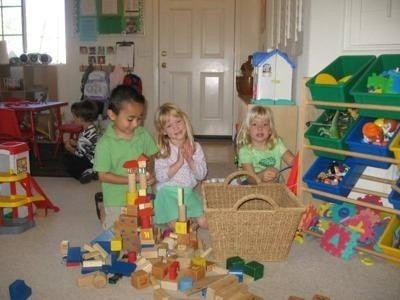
[2,75,24,91]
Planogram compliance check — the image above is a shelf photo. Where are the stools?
[53,122,82,160]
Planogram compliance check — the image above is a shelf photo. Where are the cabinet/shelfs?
[299,76,400,263]
[1,63,58,144]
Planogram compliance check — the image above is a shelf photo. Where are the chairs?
[0,107,40,161]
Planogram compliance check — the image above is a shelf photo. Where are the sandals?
[94,192,103,220]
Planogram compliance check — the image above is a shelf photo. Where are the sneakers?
[78,168,99,185]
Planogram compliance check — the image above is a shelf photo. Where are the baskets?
[201,169,306,265]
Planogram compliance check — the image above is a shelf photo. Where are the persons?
[80,46,115,64]
[91,83,160,233]
[57,97,105,185]
[150,102,210,234]
[124,16,138,33]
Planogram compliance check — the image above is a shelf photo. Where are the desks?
[0,101,68,162]
